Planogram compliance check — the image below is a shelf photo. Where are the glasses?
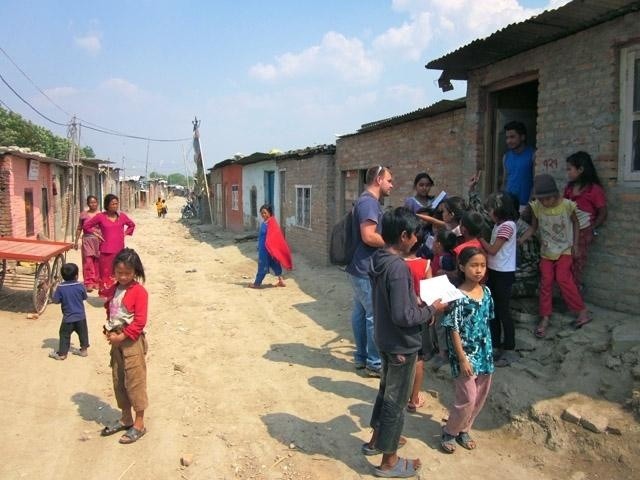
[373,165,384,180]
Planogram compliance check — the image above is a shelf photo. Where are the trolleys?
[0,235,74,316]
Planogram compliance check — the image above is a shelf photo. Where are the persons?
[161,200,168,218]
[155,197,163,217]
[502,121,536,215]
[345,165,393,379]
[404,151,606,367]
[74,196,101,292]
[361,207,448,479]
[402,221,435,412]
[97,248,152,444]
[441,246,495,452]
[49,263,90,360]
[83,194,136,302]
[249,205,294,289]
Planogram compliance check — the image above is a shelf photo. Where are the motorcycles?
[181,201,195,219]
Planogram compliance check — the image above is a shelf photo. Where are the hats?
[531,174,559,199]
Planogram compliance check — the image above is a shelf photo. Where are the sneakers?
[369,367,383,378]
[355,363,365,369]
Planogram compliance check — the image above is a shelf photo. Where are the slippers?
[457,432,475,449]
[573,315,592,328]
[374,457,419,477]
[492,348,520,366]
[407,394,424,411]
[248,285,260,288]
[74,348,86,356]
[440,421,456,453]
[535,322,547,338]
[120,427,146,443]
[272,283,286,287]
[361,438,403,455]
[102,419,134,436]
[431,355,449,371]
[49,351,60,360]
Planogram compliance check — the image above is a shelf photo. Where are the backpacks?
[329,196,382,267]
